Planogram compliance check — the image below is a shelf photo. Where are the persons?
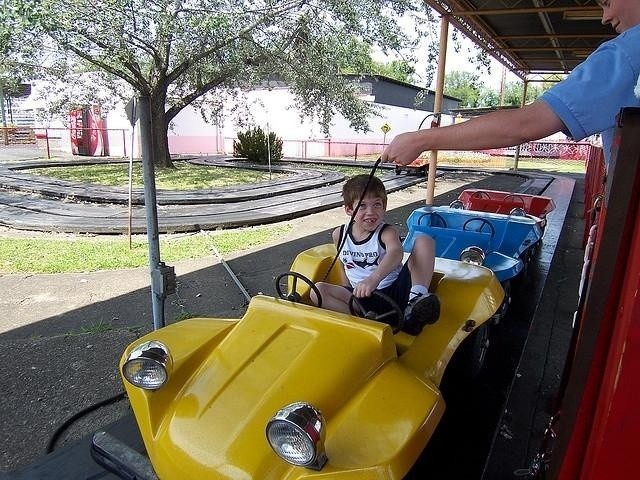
[310,174,440,336]
[380,0,640,175]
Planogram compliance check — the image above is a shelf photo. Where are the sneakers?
[403,292,440,336]
